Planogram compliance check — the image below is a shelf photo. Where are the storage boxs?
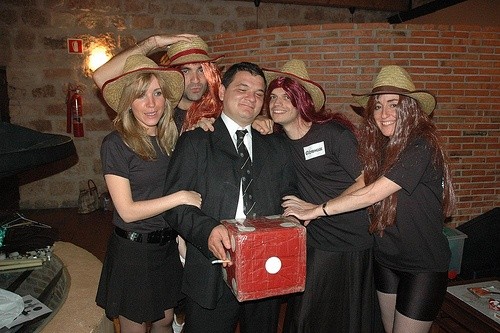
[443,225,468,274]
[218,215,307,302]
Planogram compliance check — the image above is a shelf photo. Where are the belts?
[113,226,178,244]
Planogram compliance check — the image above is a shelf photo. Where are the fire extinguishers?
[70,83,85,137]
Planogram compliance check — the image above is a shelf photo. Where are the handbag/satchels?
[77,179,100,215]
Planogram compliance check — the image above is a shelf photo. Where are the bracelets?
[322,203,329,216]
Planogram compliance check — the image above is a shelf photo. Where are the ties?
[236,129,256,219]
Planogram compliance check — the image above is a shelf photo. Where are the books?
[470,285,500,299]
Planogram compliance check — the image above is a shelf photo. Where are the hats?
[262,59,326,113]
[351,65,438,116]
[159,36,226,69]
[101,54,185,114]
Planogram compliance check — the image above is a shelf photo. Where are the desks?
[433,278,500,333]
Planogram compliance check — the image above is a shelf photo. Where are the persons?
[281,65,452,333]
[162,61,300,333]
[95,55,202,333]
[193,58,387,333]
[94,33,275,266]
